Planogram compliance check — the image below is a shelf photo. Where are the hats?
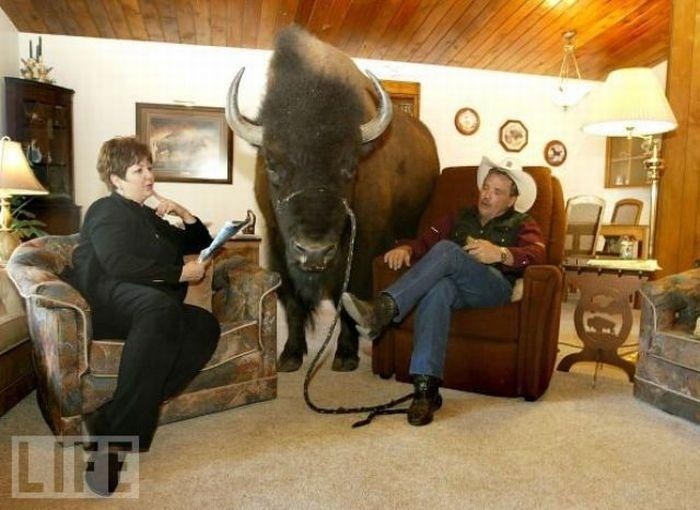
[475,152,540,215]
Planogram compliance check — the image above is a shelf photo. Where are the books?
[587,258,661,270]
[198,218,247,264]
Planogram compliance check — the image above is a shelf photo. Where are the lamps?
[1,135,49,231]
[581,65,678,185]
[551,28,589,111]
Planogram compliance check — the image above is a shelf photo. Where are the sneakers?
[80,409,107,451]
[84,446,129,498]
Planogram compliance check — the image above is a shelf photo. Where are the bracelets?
[501,246,508,264]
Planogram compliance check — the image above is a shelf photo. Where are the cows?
[225,22,440,374]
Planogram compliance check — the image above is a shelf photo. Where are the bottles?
[619,234,638,262]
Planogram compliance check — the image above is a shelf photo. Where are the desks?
[553,253,663,382]
[566,222,650,310]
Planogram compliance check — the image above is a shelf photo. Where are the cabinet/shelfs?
[5,75,83,234]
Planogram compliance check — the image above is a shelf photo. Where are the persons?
[59,136,220,496]
[342,157,547,426]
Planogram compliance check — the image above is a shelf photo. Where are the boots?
[339,291,398,343]
[406,373,445,428]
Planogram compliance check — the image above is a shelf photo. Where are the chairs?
[7,229,284,447]
[604,197,645,255]
[562,194,605,303]
[634,264,700,425]
[369,163,566,404]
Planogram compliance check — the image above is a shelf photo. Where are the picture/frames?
[453,105,481,136]
[133,101,235,188]
[497,119,529,153]
[544,139,568,166]
